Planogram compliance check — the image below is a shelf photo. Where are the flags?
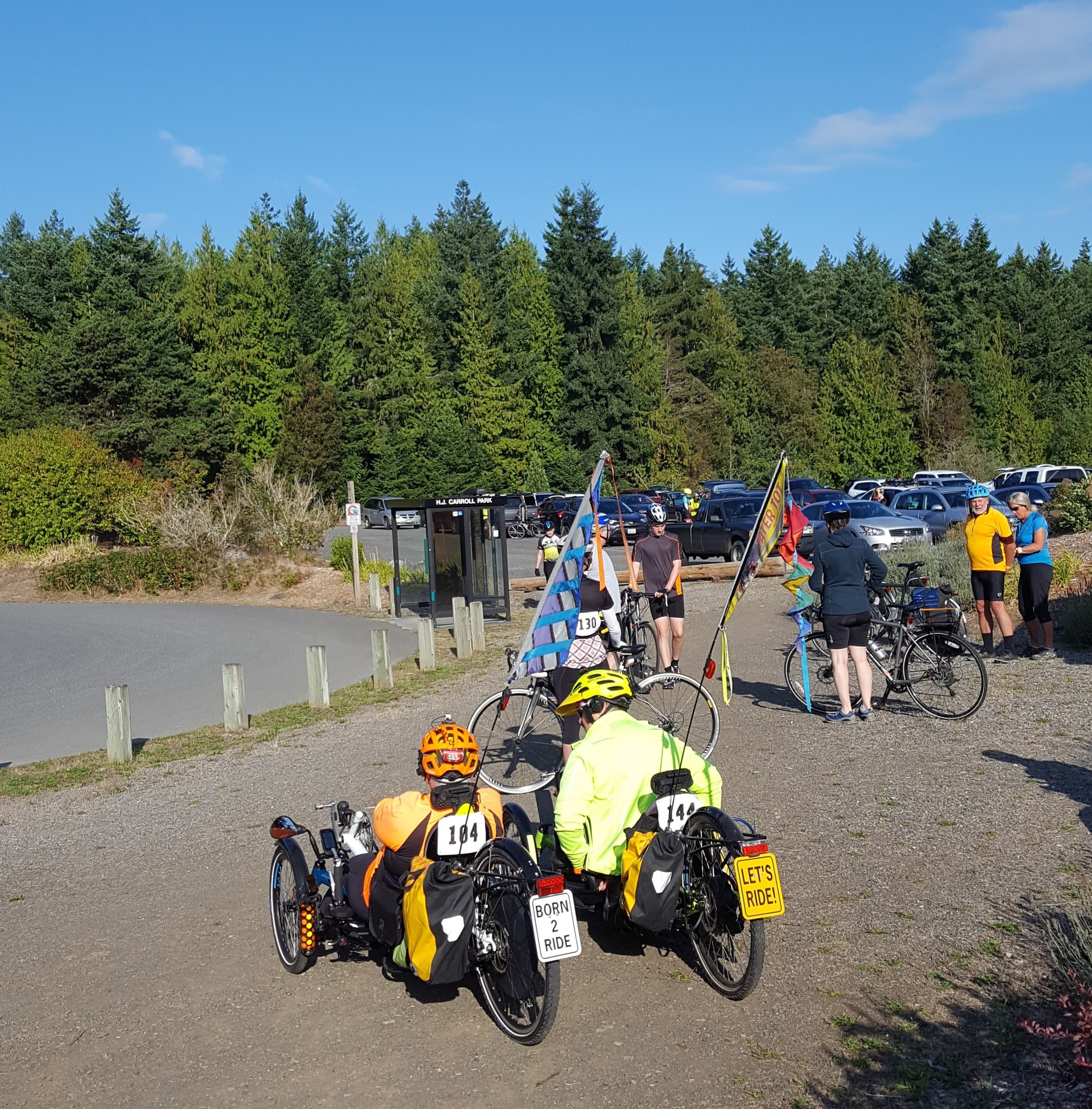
[508,450,611,680]
[718,451,788,630]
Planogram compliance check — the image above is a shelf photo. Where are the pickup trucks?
[665,497,815,567]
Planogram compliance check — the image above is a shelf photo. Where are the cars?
[799,500,933,553]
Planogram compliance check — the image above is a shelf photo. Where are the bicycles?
[508,511,547,539]
[466,641,722,795]
[620,589,668,694]
[806,560,968,664]
[783,603,988,720]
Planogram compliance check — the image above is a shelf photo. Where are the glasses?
[1012,505,1019,511]
[602,528,612,534]
[575,703,586,718]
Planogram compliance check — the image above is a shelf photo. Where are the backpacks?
[911,584,966,656]
[620,812,689,932]
[392,855,474,988]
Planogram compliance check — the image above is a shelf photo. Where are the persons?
[553,669,724,893]
[873,488,886,506]
[546,536,628,796]
[628,503,686,688]
[682,487,710,522]
[1006,491,1058,661]
[535,521,561,583]
[289,724,504,947]
[583,513,623,671]
[963,483,1019,663]
[809,499,887,724]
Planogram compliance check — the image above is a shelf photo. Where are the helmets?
[555,669,634,717]
[684,488,692,494]
[646,503,669,523]
[557,533,594,556]
[419,723,479,782]
[544,521,555,531]
[591,511,610,534]
[965,484,990,501]
[822,500,851,521]
[694,493,700,499]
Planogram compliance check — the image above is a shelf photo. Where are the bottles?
[867,639,888,660]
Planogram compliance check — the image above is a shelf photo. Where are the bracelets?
[1005,566,1012,569]
[628,584,634,589]
[1015,547,1022,554]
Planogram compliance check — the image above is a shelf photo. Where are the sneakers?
[1018,644,1043,658]
[968,650,996,661]
[996,651,1019,664]
[825,709,856,723]
[663,679,676,689]
[1031,649,1057,660]
[853,704,877,721]
[671,664,682,683]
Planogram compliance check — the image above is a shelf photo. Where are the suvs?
[1001,464,1090,489]
[889,485,1021,541]
[537,464,1055,548]
[990,481,1062,511]
[362,496,422,530]
[432,487,557,535]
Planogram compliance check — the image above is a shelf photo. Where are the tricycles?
[269,782,582,1046]
[497,768,784,1001]
